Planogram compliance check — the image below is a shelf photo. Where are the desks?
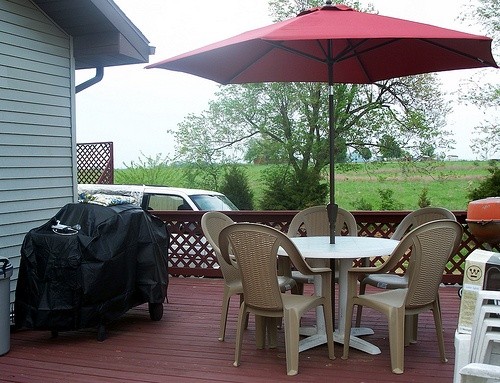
[277,236,401,355]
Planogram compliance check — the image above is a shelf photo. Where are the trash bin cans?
[0,257,13,357]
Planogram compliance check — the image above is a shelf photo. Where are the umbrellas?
[144,0,500,332]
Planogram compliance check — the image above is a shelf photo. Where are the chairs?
[218,223,336,375]
[355,208,457,344]
[177,198,193,210]
[287,205,357,328]
[201,211,300,348]
[454,289,500,383]
[340,219,463,374]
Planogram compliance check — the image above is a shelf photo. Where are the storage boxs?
[457,248,500,335]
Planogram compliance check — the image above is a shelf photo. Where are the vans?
[78,184,248,278]
